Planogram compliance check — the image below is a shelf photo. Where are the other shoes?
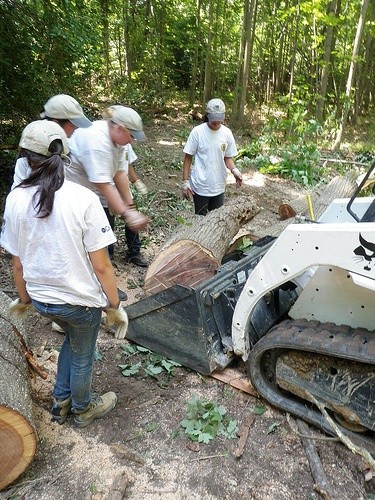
[126,249,148,266]
[117,288,127,301]
[108,252,115,265]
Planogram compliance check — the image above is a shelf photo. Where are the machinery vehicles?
[99,161,375,458]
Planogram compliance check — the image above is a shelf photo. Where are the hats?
[107,106,148,143]
[19,119,69,158]
[44,94,93,129]
[206,99,226,122]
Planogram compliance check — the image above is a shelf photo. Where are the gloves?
[122,210,148,233]
[131,179,148,201]
[231,168,243,188]
[181,180,196,201]
[6,299,35,320]
[127,204,137,212]
[106,301,129,339]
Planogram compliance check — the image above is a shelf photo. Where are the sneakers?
[50,395,70,424]
[73,391,117,427]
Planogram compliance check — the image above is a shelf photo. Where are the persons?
[184,98,243,216]
[100,144,150,266]
[68,105,148,231]
[11,94,94,334]
[0,120,129,428]
